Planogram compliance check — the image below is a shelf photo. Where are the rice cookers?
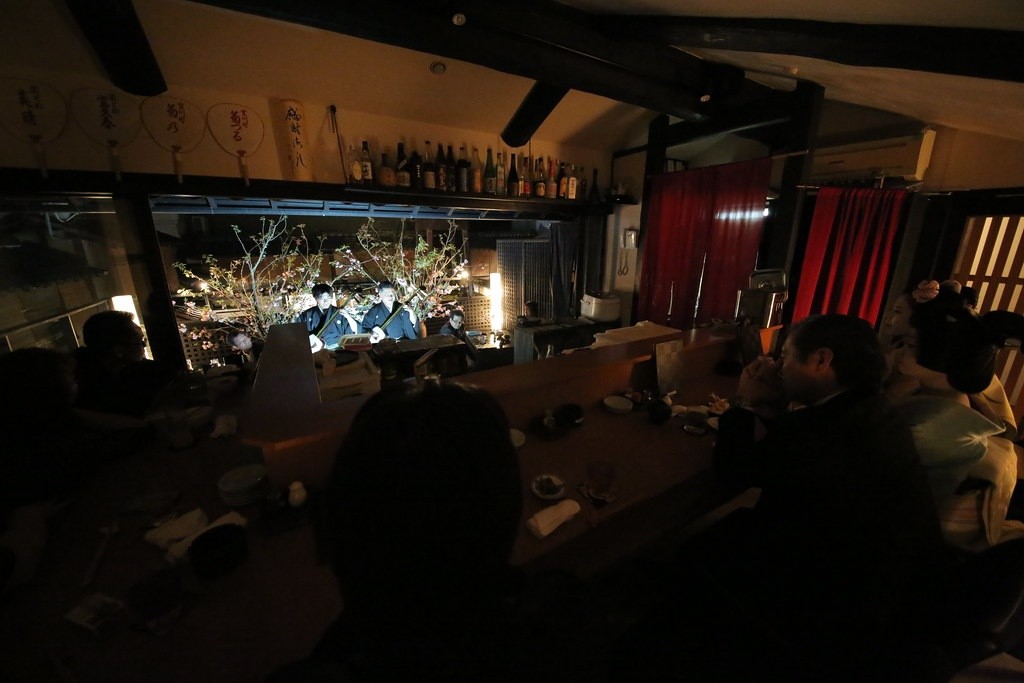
[580,291,621,322]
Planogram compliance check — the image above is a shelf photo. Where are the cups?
[319,349,336,377]
[684,411,709,436]
[587,460,616,499]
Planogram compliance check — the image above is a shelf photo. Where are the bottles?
[346,140,600,207]
[624,376,681,411]
[288,481,307,507]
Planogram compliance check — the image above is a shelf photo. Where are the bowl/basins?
[188,523,248,580]
[180,406,213,428]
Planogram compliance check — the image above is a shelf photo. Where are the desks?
[466,329,515,368]
[14,351,808,683]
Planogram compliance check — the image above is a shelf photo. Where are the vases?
[417,321,427,339]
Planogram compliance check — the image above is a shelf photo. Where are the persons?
[0,311,185,478]
[524,301,538,318]
[227,331,264,364]
[264,280,1024,683]
[441,310,467,343]
[296,283,354,353]
[361,281,421,342]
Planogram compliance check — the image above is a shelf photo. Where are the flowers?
[170,215,468,350]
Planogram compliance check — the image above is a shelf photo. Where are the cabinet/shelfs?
[514,316,623,364]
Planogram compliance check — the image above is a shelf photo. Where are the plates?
[510,428,526,449]
[531,474,565,500]
[217,464,269,508]
[604,396,634,414]
